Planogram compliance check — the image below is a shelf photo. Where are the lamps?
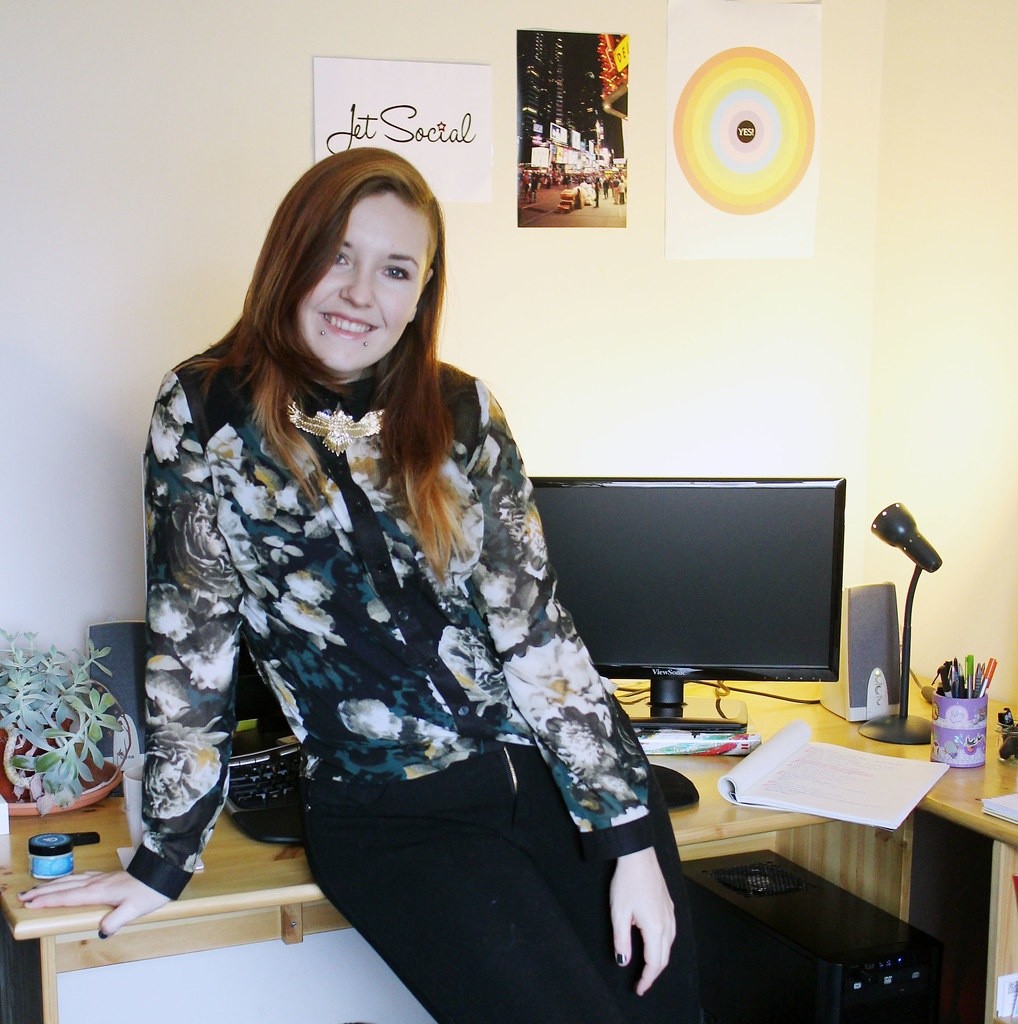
[859,502,943,745]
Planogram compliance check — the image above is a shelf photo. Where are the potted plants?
[0,626,134,820]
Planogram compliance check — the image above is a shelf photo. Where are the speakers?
[821,584,902,718]
[86,621,149,793]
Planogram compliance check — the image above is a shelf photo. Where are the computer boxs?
[676,849,946,1021]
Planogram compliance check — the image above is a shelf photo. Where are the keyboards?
[224,757,305,844]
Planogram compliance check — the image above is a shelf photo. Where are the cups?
[124,767,152,856]
[930,688,990,768]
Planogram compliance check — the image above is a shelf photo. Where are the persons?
[517,167,627,205]
[19,146,707,1024]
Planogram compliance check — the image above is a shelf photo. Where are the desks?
[0,674,1017,1024]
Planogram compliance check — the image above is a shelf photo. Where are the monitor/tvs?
[526,477,845,733]
[219,642,291,761]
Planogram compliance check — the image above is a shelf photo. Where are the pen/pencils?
[931,655,997,698]
